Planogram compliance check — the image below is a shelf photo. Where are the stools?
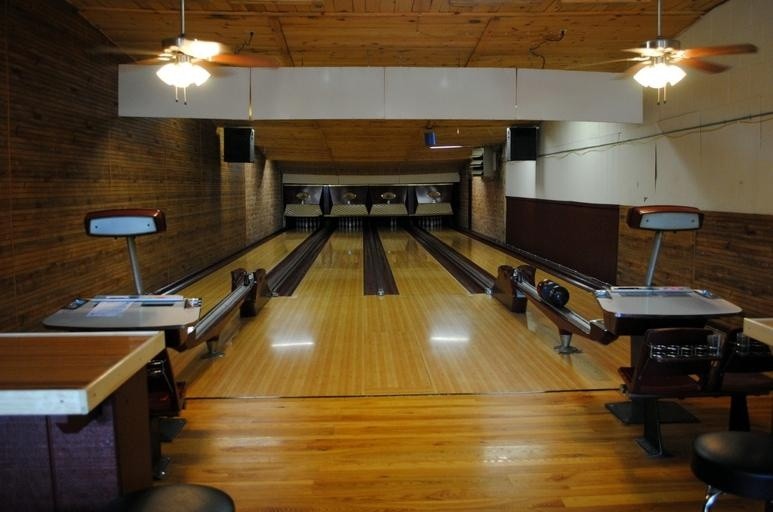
[690,430,773,512]
[112,483,235,512]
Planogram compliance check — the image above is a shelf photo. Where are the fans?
[83,0,281,79]
[561,0,757,82]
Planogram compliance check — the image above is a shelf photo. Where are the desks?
[743,318,773,346]
[592,287,743,424]
[41,295,202,442]
[0,330,166,512]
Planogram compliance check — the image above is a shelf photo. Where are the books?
[86,301,132,317]
[693,290,724,302]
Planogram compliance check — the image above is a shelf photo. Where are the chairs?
[145,359,189,481]
[617,327,773,457]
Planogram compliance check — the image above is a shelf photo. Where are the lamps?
[156,63,211,106]
[633,63,686,105]
